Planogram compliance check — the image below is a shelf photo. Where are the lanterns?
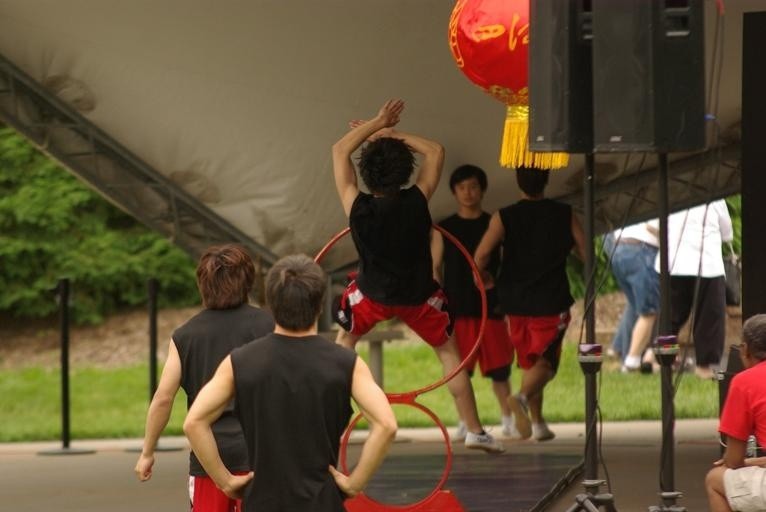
[447,0,570,171]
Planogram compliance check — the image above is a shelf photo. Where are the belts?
[613,239,641,245]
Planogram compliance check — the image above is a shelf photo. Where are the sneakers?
[503,427,513,438]
[465,432,506,453]
[507,395,532,439]
[622,356,641,373]
[457,422,468,440]
[607,349,619,360]
[534,429,555,440]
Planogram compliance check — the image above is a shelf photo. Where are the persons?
[470,162,589,442]
[603,218,660,376]
[651,198,733,379]
[703,313,765,512]
[329,96,508,456]
[133,244,276,512]
[180,254,399,512]
[428,163,521,443]
